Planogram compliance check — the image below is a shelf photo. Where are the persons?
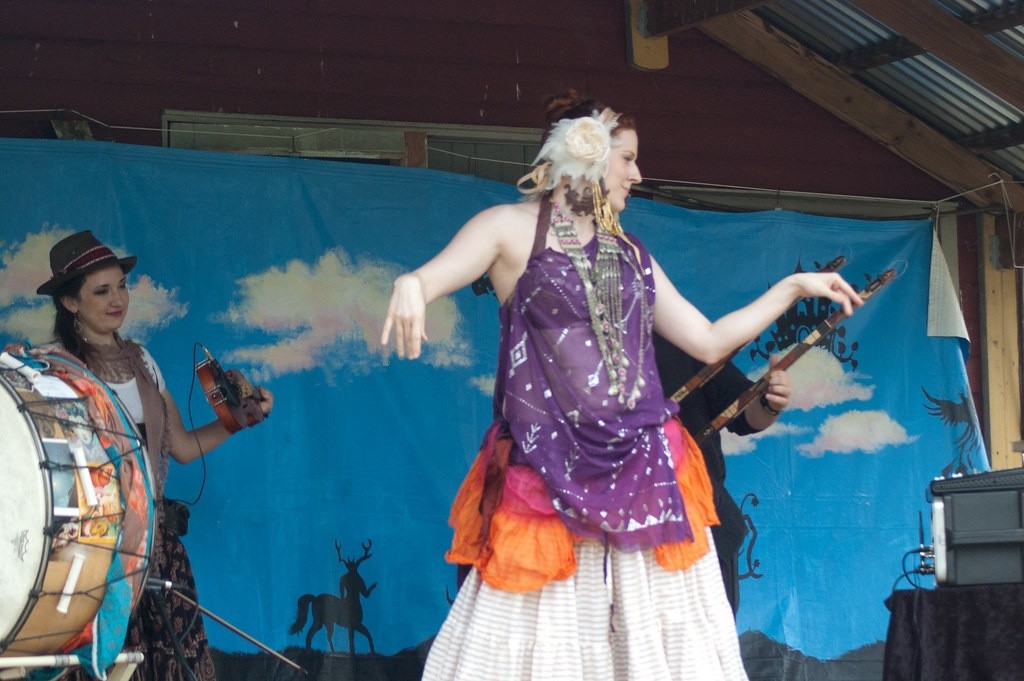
[651,330,793,622]
[36,230,273,681]
[381,89,864,681]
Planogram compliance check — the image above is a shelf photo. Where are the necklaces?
[549,201,651,410]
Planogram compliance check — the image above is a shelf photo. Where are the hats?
[37,230,137,295]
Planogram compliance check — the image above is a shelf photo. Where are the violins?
[195,356,267,434]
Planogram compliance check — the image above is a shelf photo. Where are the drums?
[0,344,156,681]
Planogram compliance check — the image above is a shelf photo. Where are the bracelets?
[759,395,780,417]
